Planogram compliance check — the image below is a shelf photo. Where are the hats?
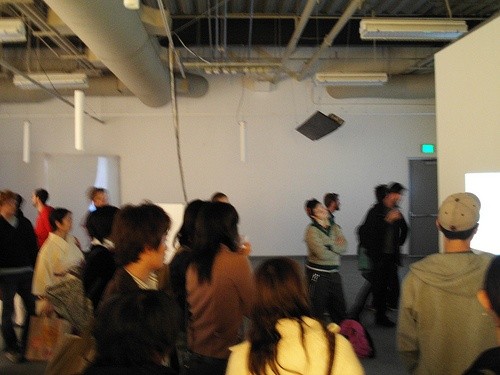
[438,193,481,232]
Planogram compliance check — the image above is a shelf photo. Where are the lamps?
[0,18,25,43]
[14,73,89,90]
[359,18,468,41]
[314,73,388,86]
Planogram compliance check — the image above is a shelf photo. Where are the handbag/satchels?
[26,312,72,362]
[43,332,99,375]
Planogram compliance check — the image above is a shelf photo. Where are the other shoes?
[5,350,22,362]
[374,318,396,328]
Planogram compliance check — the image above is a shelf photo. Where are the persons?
[0,181,500,375]
[88,199,171,375]
[224,258,366,375]
[395,192,500,375]
[302,198,349,322]
[31,208,94,337]
